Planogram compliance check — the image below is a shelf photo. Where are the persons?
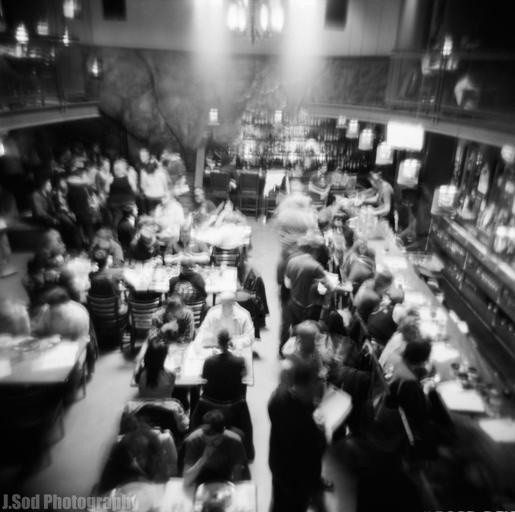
[1,71,514,511]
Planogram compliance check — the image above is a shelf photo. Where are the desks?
[71,255,238,296]
[175,340,253,389]
[103,473,257,512]
[0,335,92,437]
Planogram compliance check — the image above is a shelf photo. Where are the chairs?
[126,398,256,462]
[211,246,266,338]
[88,295,205,351]
